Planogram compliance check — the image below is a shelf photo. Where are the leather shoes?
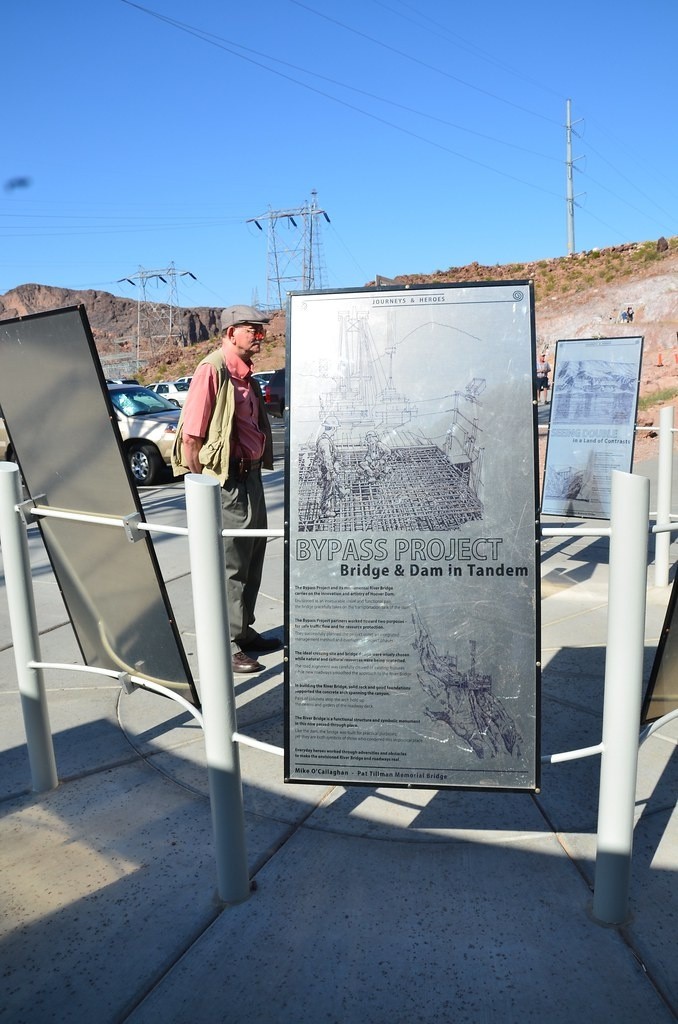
[237,633,283,654]
[230,650,259,673]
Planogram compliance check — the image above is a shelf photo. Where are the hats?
[221,305,271,331]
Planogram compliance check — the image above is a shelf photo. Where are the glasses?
[234,327,267,341]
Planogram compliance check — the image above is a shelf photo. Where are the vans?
[134,383,190,409]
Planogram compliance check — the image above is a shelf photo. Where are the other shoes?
[545,401,549,404]
[538,400,541,403]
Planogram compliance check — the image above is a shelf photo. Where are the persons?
[621,307,634,323]
[170,306,284,674]
[537,354,551,404]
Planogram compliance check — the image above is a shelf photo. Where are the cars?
[107,382,186,486]
[175,376,193,388]
[261,368,286,424]
[105,376,141,387]
[253,375,269,401]
[250,371,275,384]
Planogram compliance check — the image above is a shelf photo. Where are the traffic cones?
[657,354,664,367]
